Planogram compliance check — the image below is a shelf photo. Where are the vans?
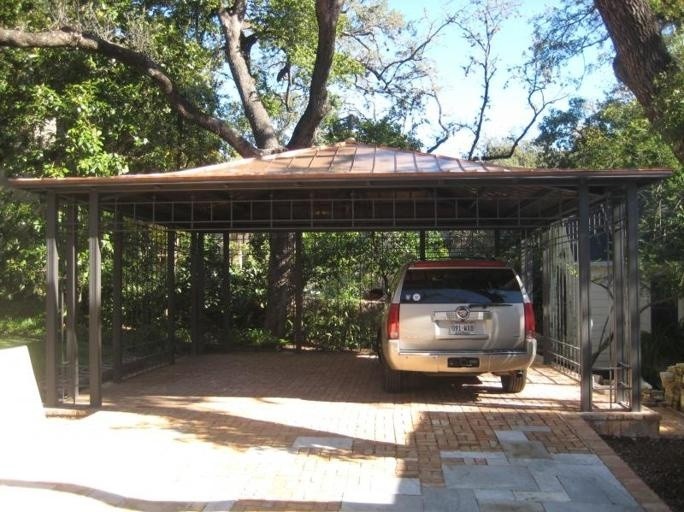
[368,261,536,393]
